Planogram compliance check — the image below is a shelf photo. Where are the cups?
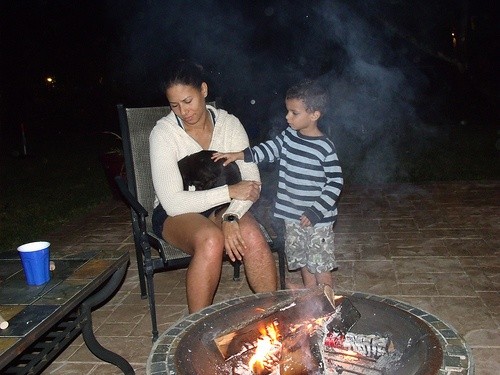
[17,241,51,286]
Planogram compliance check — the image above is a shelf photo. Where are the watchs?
[220,213,239,224]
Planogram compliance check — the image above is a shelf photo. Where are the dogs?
[179,150,241,220]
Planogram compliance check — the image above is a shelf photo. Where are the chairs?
[113,101,286,344]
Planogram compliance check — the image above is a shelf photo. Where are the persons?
[210,77,343,288]
[152,59,278,317]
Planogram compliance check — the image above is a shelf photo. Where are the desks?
[0,249,136,375]
